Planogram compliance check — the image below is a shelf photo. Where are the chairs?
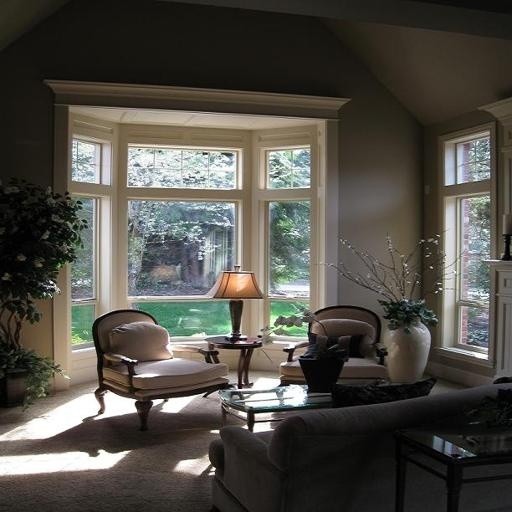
[92,310,234,432]
[279,304,388,385]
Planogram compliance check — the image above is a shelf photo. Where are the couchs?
[209,381,512,510]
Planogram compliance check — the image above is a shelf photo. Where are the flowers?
[312,225,467,334]
[275,298,341,357]
[1,175,89,406]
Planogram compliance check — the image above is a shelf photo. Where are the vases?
[3,369,28,407]
[385,317,432,385]
[298,358,348,394]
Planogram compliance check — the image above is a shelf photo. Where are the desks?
[481,259,511,381]
[204,336,262,389]
[394,423,512,511]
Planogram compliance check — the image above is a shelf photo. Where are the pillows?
[304,331,367,358]
[330,375,438,407]
[492,376,512,384]
[108,321,173,367]
[311,319,376,351]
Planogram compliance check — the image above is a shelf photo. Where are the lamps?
[214,266,265,341]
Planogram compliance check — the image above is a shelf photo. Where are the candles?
[501,214,512,234]
